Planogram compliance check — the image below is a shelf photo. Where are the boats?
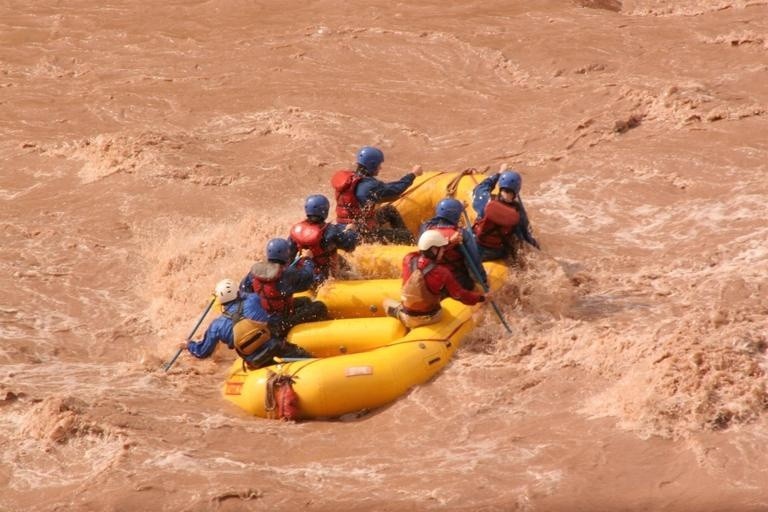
[222,171,524,418]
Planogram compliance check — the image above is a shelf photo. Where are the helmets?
[418,230,450,251]
[434,198,463,225]
[266,238,290,264]
[215,279,239,305]
[356,146,384,175]
[305,194,329,220]
[498,171,521,198]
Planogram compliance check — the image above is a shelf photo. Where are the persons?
[470,163,541,261]
[383,230,490,329]
[330,146,423,246]
[287,193,357,281]
[417,197,491,291]
[238,237,327,324]
[179,278,315,372]
